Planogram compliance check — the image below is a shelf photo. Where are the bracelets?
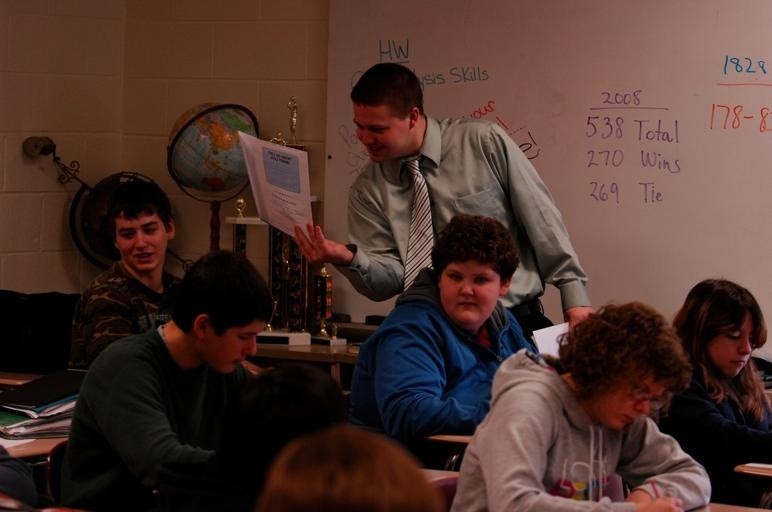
[645,478,662,499]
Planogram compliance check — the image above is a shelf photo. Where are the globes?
[167,102,260,252]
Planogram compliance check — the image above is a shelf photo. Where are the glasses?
[621,373,664,412]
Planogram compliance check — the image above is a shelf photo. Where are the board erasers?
[330,313,351,323]
[365,315,386,326]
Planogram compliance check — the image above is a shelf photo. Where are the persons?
[253,419,450,510]
[64,186,187,370]
[447,302,714,512]
[344,211,540,471]
[653,277,770,511]
[178,357,350,510]
[286,59,595,358]
[51,250,278,510]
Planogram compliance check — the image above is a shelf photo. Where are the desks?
[0,312,772,512]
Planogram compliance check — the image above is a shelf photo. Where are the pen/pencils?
[650,479,661,499]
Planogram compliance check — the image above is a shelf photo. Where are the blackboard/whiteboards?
[324,0,772,366]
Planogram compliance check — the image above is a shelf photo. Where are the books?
[235,129,315,246]
[527,322,572,362]
[1,367,89,441]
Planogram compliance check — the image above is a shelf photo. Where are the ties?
[404,159,435,293]
